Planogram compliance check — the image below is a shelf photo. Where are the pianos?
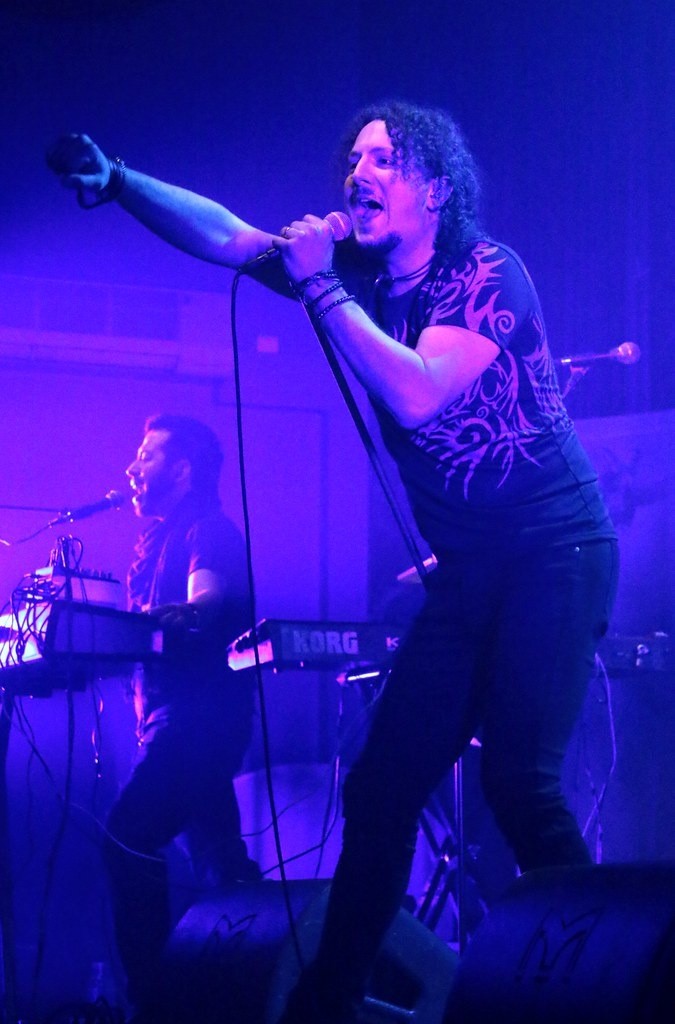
[223,614,672,676]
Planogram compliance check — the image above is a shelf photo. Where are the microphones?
[237,212,351,275]
[553,340,641,368]
[48,491,125,527]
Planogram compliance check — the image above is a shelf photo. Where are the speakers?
[438,866,675,1024]
[146,874,462,1024]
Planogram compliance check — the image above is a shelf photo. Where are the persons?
[45,101,617,1024]
[106,413,259,989]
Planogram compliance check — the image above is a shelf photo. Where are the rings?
[284,227,292,238]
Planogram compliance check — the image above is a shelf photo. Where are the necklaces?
[373,258,433,287]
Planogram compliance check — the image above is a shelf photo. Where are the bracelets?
[188,601,201,628]
[316,295,356,321]
[308,282,343,308]
[300,269,336,288]
[76,158,125,209]
